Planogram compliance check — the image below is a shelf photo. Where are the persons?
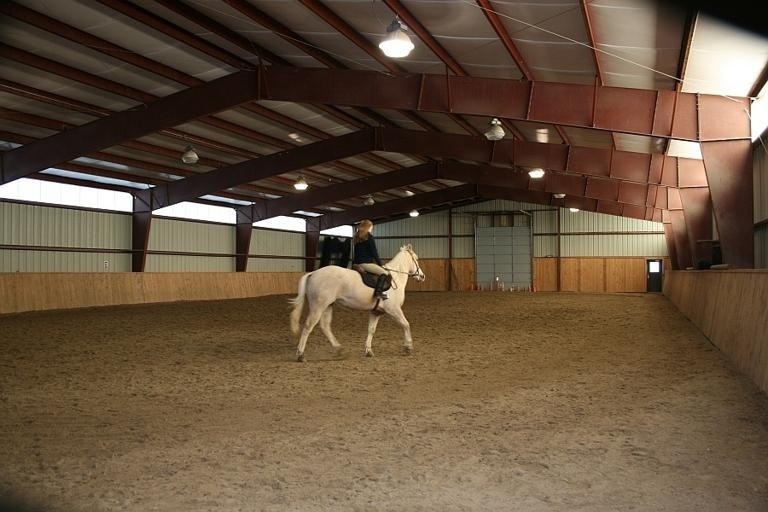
[354,220,391,300]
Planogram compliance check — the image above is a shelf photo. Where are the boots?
[374,274,387,298]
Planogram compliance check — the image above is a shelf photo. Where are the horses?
[283,243,426,362]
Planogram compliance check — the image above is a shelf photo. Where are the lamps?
[180,141,200,163]
[363,193,374,206]
[483,117,505,142]
[379,13,415,59]
[293,175,308,191]
[528,168,545,180]
[408,208,419,217]
[552,193,566,199]
